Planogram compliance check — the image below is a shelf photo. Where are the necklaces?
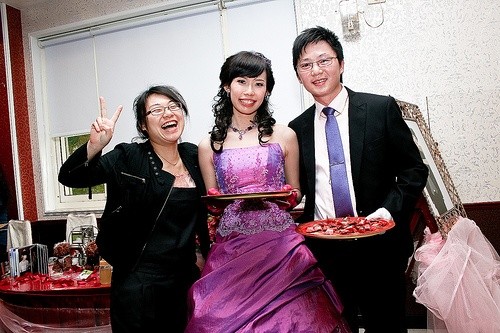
[228,122,258,140]
[153,148,180,166]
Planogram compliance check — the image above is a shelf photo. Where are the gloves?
[278,184,298,210]
[205,187,223,216]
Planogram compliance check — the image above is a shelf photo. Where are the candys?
[306,216,388,235]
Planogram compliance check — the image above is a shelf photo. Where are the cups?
[99,257,113,272]
[99,266,111,284]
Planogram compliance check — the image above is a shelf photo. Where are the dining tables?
[0,265,111,333]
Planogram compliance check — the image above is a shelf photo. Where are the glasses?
[296,56,338,72]
[144,102,183,116]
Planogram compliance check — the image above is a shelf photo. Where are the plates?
[201,190,293,199]
[295,217,395,239]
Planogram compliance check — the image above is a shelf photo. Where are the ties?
[323,106,356,218]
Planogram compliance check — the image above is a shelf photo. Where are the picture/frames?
[395,98,468,239]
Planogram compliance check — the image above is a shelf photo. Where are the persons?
[183,49,351,332]
[57,84,211,333]
[15,245,48,276]
[275,25,428,333]
[407,128,448,217]
